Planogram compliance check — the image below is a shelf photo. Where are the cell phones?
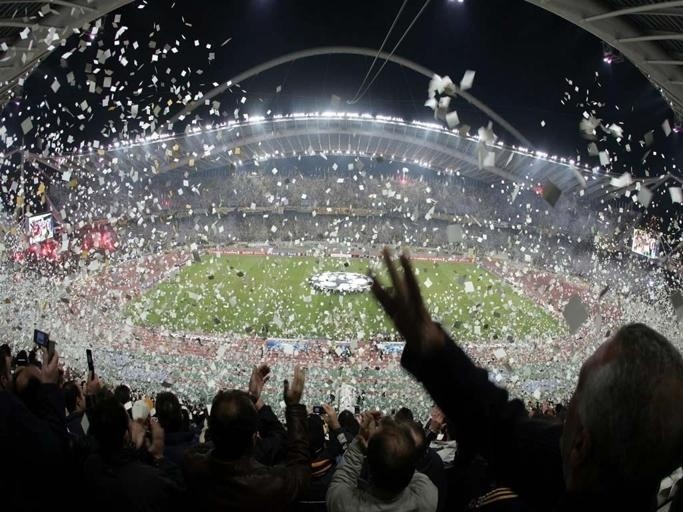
[313,406,327,414]
[86,349,94,380]
[355,406,360,415]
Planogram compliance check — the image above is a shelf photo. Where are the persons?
[361,240,682,511]
[2,333,683,512]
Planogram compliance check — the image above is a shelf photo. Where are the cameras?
[34,329,48,347]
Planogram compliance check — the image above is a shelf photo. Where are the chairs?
[309,459,332,477]
[476,488,518,508]
